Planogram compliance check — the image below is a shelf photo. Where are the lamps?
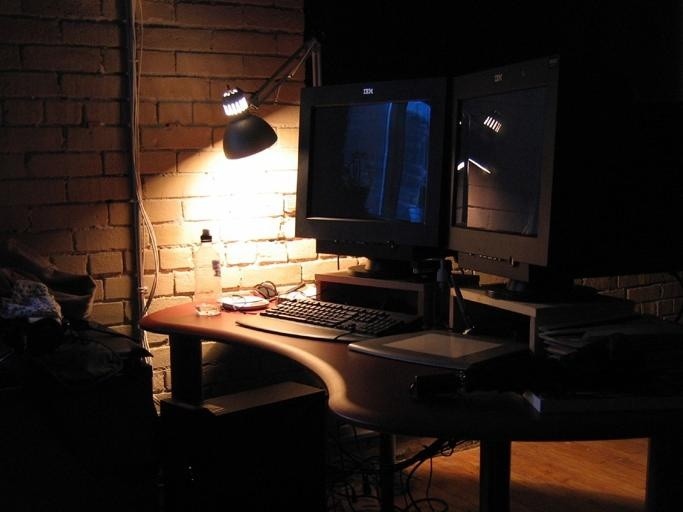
[221,31,322,159]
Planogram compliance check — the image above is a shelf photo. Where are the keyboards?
[235,296,405,344]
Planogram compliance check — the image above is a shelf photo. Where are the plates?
[217,296,270,310]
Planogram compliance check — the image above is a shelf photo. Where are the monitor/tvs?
[298,76,445,281]
[444,56,599,300]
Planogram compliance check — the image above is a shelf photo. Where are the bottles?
[193,229,222,317]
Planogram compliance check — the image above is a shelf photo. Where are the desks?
[140,284,683,510]
